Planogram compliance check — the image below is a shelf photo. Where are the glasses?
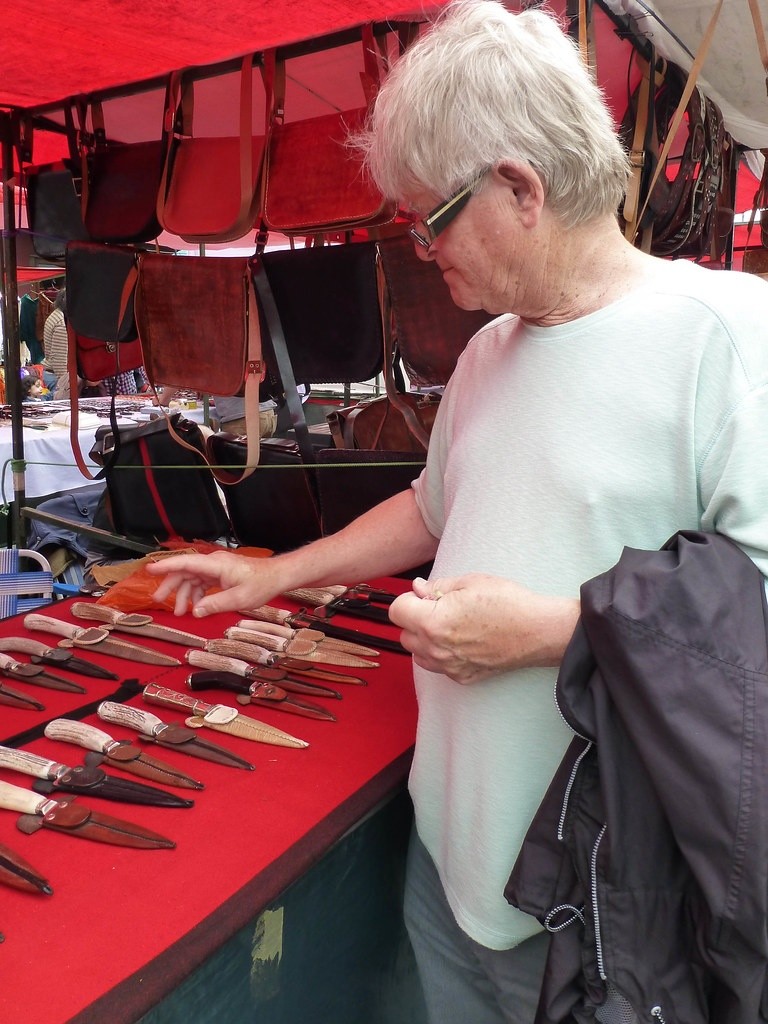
[404,158,537,247]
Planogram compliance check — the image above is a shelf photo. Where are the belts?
[618,53,737,269]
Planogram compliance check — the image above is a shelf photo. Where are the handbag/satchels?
[158,134,264,244]
[327,389,441,454]
[90,413,227,544]
[377,238,508,388]
[742,249,768,282]
[70,329,144,382]
[253,241,388,384]
[261,103,398,235]
[135,256,264,397]
[65,242,142,342]
[82,138,165,242]
[208,432,322,551]
[27,170,91,258]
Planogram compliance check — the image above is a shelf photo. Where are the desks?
[0,395,221,546]
[0,572,423,1024]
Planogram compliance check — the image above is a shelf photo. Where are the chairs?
[0,549,54,622]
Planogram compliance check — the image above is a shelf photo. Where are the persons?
[153,365,278,439]
[142,0,767,1024]
[20,287,151,401]
